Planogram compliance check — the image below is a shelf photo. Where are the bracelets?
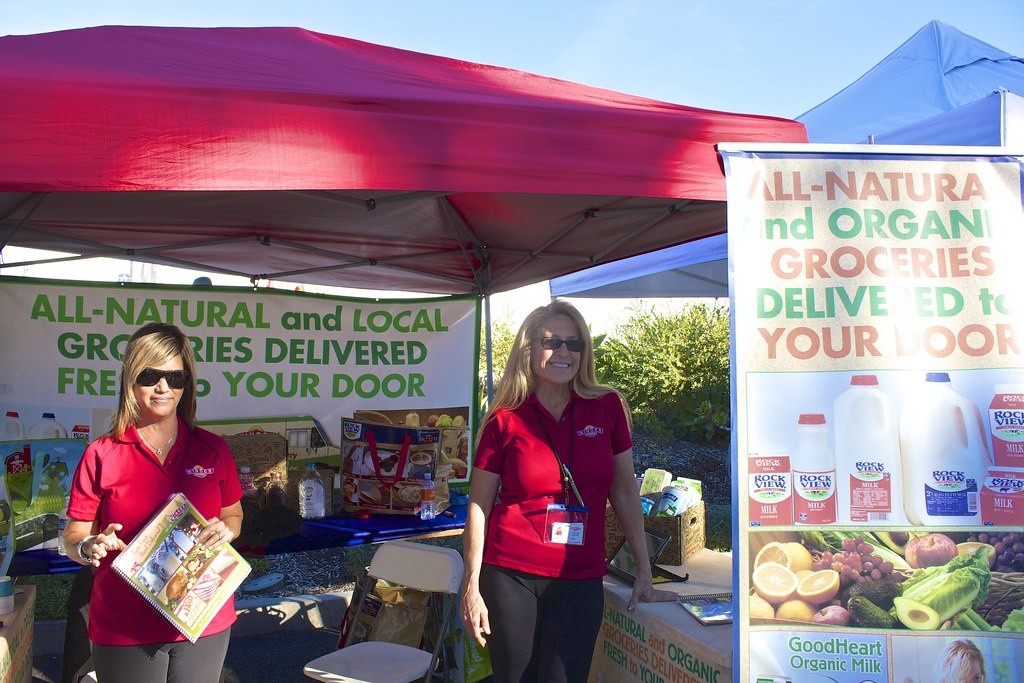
[78,535,94,563]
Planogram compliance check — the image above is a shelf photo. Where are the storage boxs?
[605,499,707,573]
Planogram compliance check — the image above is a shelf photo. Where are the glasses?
[532,336,584,353]
[136,367,193,389]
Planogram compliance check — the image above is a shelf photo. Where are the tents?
[0,27,808,409]
[550,21,1024,305]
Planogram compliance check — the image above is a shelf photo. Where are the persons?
[938,639,986,682]
[62,323,243,683]
[460,301,679,683]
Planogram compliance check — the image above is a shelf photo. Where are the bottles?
[237,464,257,524]
[0,410,25,441]
[299,464,325,520]
[265,472,286,518]
[791,413,837,526]
[58,502,70,556]
[0,576,15,615]
[28,412,68,440]
[898,373,996,527]
[420,472,436,520]
[836,374,899,523]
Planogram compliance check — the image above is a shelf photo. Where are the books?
[675,592,732,626]
[111,492,252,645]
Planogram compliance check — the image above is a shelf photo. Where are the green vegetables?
[799,530,909,569]
[888,545,1024,632]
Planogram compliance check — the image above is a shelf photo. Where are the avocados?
[868,531,915,555]
[839,579,940,630]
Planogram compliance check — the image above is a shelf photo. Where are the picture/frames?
[606,527,670,585]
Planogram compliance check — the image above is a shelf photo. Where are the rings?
[217,532,222,539]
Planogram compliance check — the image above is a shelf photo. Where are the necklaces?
[135,421,177,455]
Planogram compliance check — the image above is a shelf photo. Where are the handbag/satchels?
[335,564,431,649]
[340,416,442,516]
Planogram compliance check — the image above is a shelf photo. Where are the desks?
[586,547,731,683]
[0,584,37,683]
[7,490,470,577]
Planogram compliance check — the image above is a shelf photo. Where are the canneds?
[979,383,1023,528]
[656,485,691,517]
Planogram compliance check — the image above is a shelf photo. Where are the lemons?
[748,542,841,622]
[955,541,996,570]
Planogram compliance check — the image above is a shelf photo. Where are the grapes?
[811,535,906,585]
[961,532,1024,573]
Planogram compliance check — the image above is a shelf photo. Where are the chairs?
[304,540,464,683]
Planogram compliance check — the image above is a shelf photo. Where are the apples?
[809,605,850,626]
[905,533,958,568]
